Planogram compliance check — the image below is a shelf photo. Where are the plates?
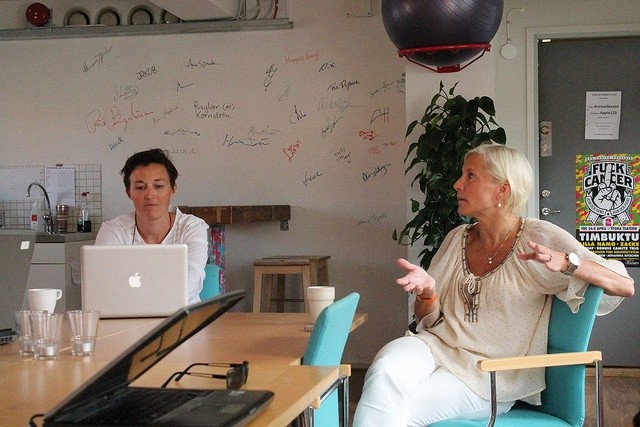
[160,9,184,25]
[64,7,93,27]
[127,5,154,28]
[94,6,121,27]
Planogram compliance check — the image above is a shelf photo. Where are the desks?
[0,312,368,427]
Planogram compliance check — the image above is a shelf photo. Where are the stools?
[261,255,332,314]
[252,258,313,315]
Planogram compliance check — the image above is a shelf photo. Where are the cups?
[13,310,50,357]
[306,285,336,324]
[29,314,63,360]
[26,287,63,316]
[66,308,101,357]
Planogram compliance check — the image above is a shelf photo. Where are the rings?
[546,254,552,262]
[416,288,424,295]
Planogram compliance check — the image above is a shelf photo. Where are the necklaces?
[132,211,171,245]
[482,218,515,264]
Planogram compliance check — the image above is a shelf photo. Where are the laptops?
[40,290,276,427]
[0,226,36,345]
[79,243,189,320]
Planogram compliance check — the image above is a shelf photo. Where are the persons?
[94,149,209,306]
[351,145,635,427]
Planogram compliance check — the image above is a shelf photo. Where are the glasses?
[160,360,251,394]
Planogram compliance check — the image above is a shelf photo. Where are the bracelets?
[417,291,437,304]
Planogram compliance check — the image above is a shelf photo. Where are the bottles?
[55,204,69,232]
[77,191,92,232]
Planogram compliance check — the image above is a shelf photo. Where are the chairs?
[425,284,606,427]
[296,292,361,427]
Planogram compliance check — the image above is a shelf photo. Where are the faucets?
[25,181,53,232]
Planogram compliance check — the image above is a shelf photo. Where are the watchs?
[560,252,581,276]
